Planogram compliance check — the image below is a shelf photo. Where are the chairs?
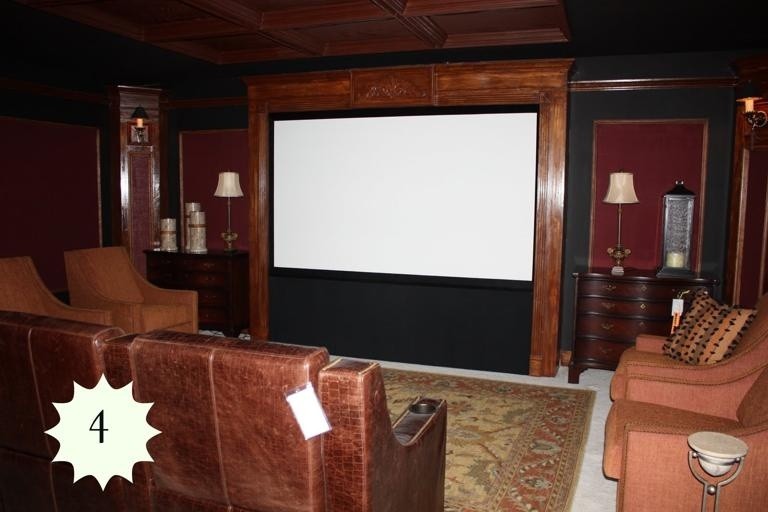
[105,328,447,512]
[610,294,768,402]
[0,257,112,328]
[0,309,129,511]
[602,359,768,512]
[66,245,200,337]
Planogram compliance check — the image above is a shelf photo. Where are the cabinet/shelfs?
[567,266,719,383]
[143,249,250,338]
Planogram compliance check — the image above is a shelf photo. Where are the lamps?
[185,202,202,249]
[189,213,208,252]
[602,173,639,276]
[158,217,177,251]
[688,431,747,512]
[214,169,244,253]
[131,103,151,142]
[734,78,767,132]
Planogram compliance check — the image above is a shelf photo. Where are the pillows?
[663,291,757,364]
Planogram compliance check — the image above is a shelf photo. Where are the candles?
[668,252,684,268]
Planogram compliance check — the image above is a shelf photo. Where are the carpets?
[380,366,596,512]
